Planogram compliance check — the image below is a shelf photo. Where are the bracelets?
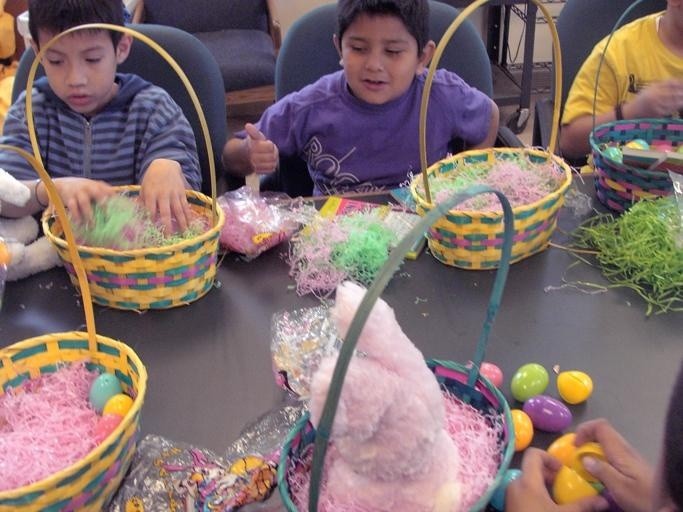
[33,177,47,209]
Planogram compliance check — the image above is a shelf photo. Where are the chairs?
[129,0,282,119]
[11,22,234,204]
[532,0,668,170]
[259,1,526,199]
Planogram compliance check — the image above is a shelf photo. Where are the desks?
[0,172,683,511]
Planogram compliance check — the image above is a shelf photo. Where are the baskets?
[408,0,573,272]
[25,22,225,316]
[588,0,683,214]
[277,183,513,512]
[0,146,148,512]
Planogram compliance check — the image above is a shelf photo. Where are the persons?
[0,1,204,236]
[553,0,683,169]
[219,0,501,200]
[502,358,681,511]
